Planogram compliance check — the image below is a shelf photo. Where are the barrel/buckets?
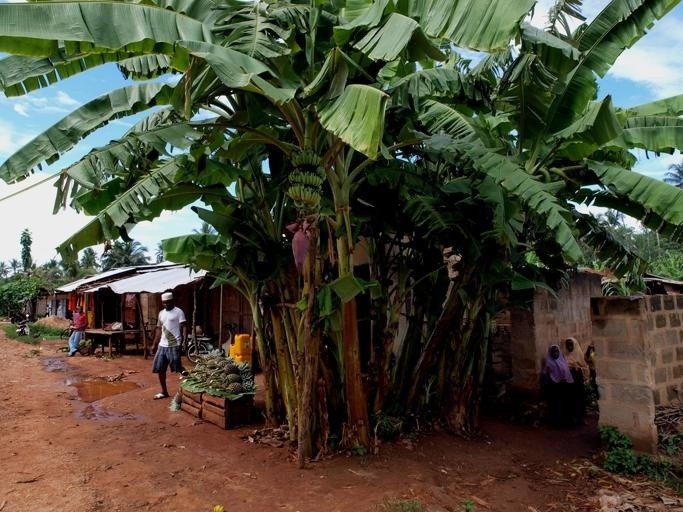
[230,334,251,367]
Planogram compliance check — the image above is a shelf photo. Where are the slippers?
[153,392,169,400]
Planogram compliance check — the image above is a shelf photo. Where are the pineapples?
[192,355,255,393]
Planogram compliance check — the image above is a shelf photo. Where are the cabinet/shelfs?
[180,382,255,428]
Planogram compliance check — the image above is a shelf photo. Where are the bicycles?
[60,323,72,340]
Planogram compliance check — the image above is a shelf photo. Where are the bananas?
[288,154,322,204]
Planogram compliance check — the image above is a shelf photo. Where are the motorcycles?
[16,320,29,336]
[187,324,237,362]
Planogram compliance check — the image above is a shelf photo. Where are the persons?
[583,340,600,407]
[564,336,587,428]
[43,304,51,317]
[149,292,191,400]
[540,343,576,429]
[66,305,88,356]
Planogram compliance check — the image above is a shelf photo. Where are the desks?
[83,328,123,358]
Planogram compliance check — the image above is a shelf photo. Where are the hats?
[161,292,174,301]
